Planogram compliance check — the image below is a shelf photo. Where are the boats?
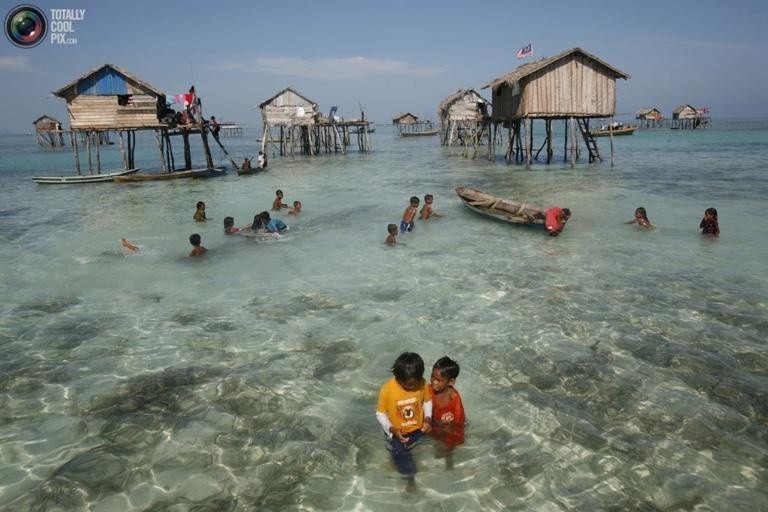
[109,165,226,184]
[454,186,568,227]
[31,167,141,185]
[582,125,640,139]
[254,125,440,145]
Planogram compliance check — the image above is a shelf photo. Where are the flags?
[516,43,533,59]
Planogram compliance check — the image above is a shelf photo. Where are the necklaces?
[400,196,419,233]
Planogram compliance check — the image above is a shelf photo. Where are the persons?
[384,224,398,245]
[376,352,433,494]
[428,356,465,472]
[184,86,220,140]
[419,194,441,221]
[549,208,570,236]
[700,208,721,239]
[620,207,652,229]
[257,151,265,168]
[122,190,302,257]
[242,157,254,170]
